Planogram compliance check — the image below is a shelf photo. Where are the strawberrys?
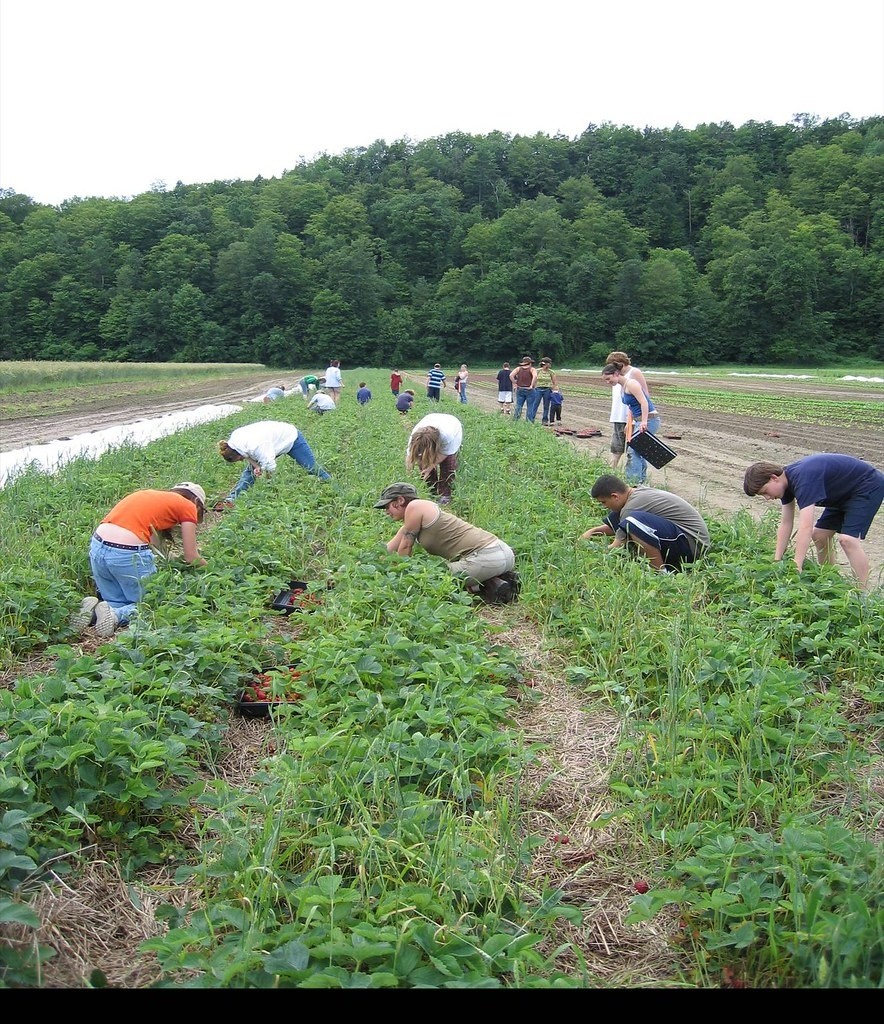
[635,881,647,893]
[526,680,532,686]
[680,921,687,927]
[720,967,744,988]
[216,502,233,508]
[554,427,599,436]
[245,669,302,701]
[554,835,570,844]
[287,588,323,606]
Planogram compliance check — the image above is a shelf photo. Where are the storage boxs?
[273,580,322,615]
[626,430,678,469]
[238,664,302,716]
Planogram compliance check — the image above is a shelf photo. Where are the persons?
[396,389,414,414]
[266,386,285,401]
[496,362,516,415]
[531,357,556,426]
[356,382,372,404]
[602,361,660,486]
[70,482,208,637]
[606,352,649,468]
[550,386,564,426]
[307,390,335,414]
[406,413,463,505]
[458,364,468,404]
[324,360,345,402]
[425,364,446,402]
[583,475,710,575]
[216,421,330,511]
[509,357,537,421]
[374,482,522,605]
[300,375,326,398]
[743,453,884,590]
[390,369,402,396]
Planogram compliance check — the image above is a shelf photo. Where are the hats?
[519,356,535,366]
[171,482,205,523]
[373,482,418,509]
[405,389,415,396]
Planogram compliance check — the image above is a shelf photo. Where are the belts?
[93,532,149,551]
[634,413,659,423]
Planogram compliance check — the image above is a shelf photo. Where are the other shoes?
[69,596,100,632]
[499,571,521,601]
[95,601,116,639]
[213,501,234,511]
[438,495,451,505]
[479,578,509,608]
